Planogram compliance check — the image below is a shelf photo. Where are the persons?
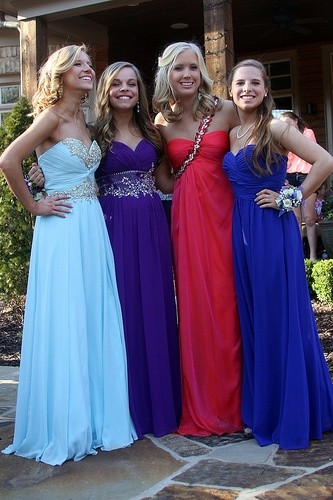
[152,42,246,438]
[0,43,138,465]
[316,183,330,220]
[280,112,318,261]
[27,62,180,440]
[222,57,333,448]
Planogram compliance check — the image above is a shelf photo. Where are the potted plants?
[313,187,333,260]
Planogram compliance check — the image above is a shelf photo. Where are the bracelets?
[275,184,306,213]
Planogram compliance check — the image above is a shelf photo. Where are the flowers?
[275,185,306,219]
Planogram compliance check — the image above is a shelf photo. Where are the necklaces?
[236,118,260,140]
